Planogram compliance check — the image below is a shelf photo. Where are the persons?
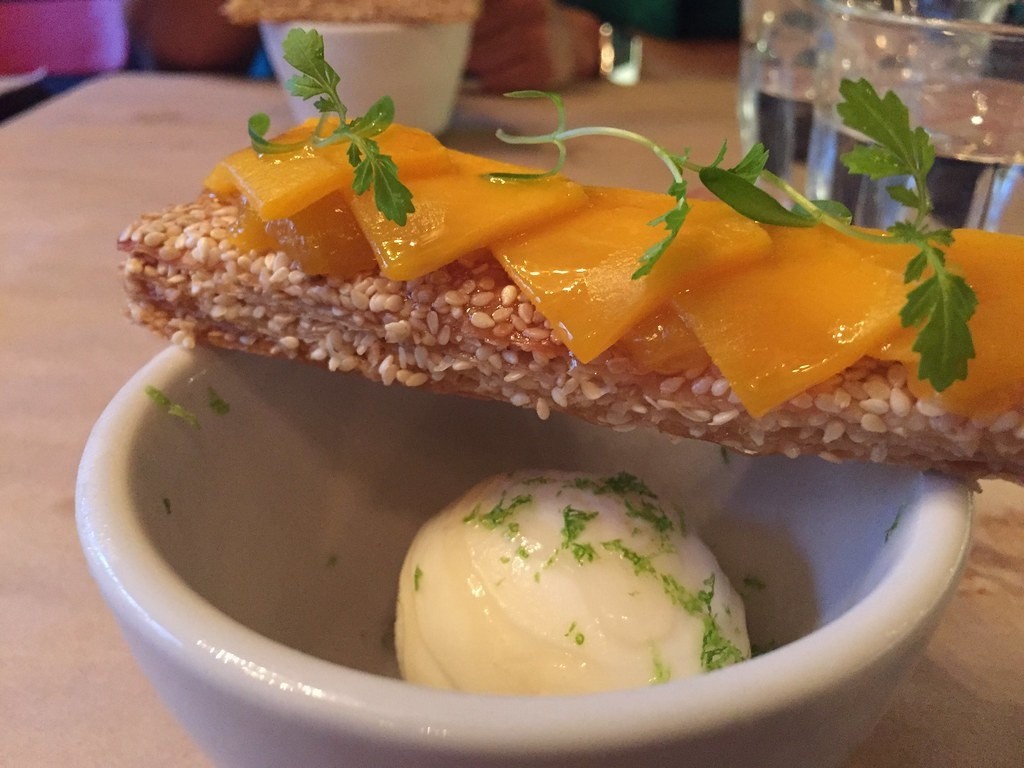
[136,0,740,97]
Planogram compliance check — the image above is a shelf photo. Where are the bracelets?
[597,16,644,86]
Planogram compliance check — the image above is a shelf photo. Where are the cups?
[738,2,835,193]
[805,0,1024,232]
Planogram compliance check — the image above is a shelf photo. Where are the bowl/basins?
[72,334,970,767]
[258,19,473,140]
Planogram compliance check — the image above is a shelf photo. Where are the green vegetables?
[245,25,977,395]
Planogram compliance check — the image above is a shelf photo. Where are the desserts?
[391,465,754,697]
